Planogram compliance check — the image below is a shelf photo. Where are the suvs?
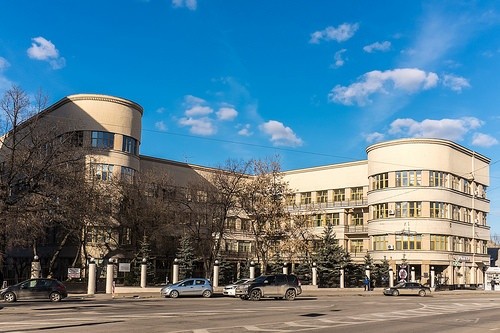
[236,274,302,301]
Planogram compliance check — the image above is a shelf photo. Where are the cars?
[382,282,431,297]
[160,278,213,298]
[0,278,68,303]
[223,278,254,298]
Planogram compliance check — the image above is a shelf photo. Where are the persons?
[363,275,371,291]
[490,278,496,290]
